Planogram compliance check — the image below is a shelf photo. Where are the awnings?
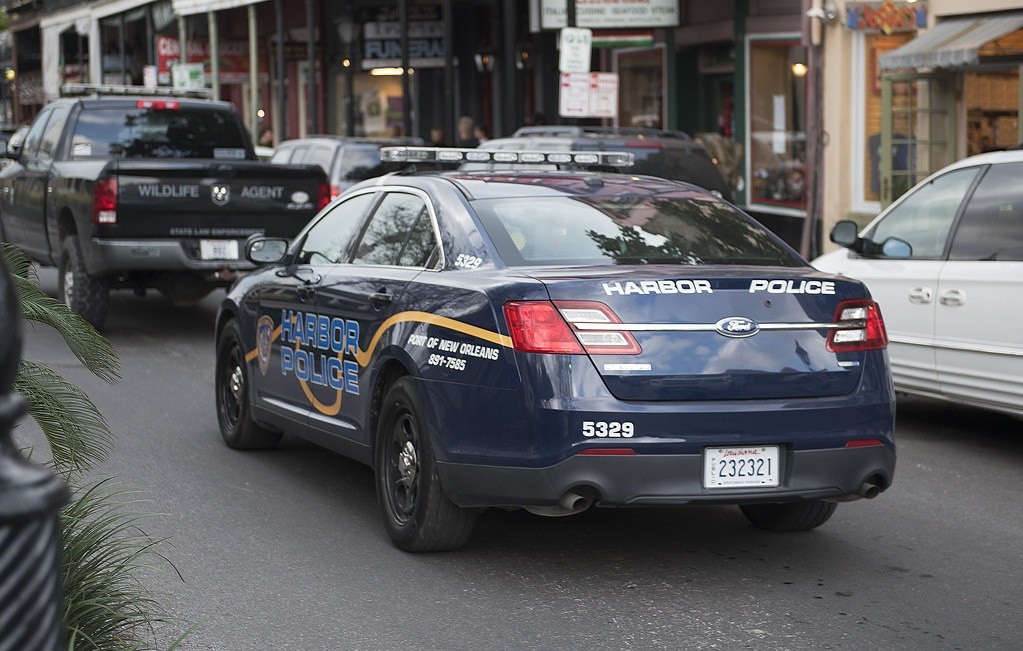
[879,9,1023,70]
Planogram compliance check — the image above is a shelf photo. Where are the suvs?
[459,126,741,206]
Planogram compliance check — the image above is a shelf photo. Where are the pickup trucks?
[0,84,334,332]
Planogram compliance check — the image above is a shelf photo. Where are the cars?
[805,151,1023,419]
[7,118,33,156]
[213,146,899,554]
[267,130,444,201]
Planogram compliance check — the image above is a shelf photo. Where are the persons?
[259,129,272,148]
[426,114,488,148]
